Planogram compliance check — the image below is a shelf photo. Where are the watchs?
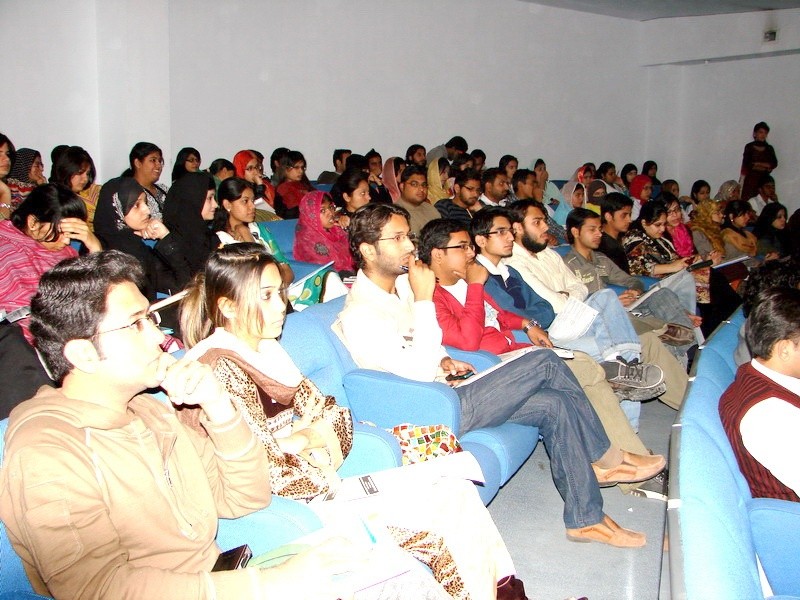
[523,319,538,333]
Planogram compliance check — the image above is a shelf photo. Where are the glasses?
[289,166,306,171]
[84,312,162,340]
[405,181,428,188]
[477,229,516,235]
[460,185,482,193]
[437,244,475,251]
[667,207,680,215]
[320,205,336,215]
[245,165,262,171]
[186,158,199,163]
[364,232,415,242]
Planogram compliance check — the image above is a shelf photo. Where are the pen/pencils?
[399,265,440,280]
[360,519,377,544]
[637,295,641,298]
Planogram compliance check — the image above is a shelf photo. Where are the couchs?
[0,218,539,600]
[657,305,800,600]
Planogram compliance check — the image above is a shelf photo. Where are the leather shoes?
[591,451,666,488]
[565,513,645,548]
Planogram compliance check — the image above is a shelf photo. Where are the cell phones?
[445,370,473,380]
[211,543,247,572]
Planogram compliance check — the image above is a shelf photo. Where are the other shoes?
[496,576,530,600]
[658,323,694,347]
[612,383,667,402]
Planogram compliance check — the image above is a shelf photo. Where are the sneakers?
[630,471,669,497]
[607,356,663,388]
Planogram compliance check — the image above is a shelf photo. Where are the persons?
[0,133,102,233]
[740,122,778,203]
[1,251,450,600]
[163,136,800,432]
[0,182,183,350]
[175,243,527,600]
[342,201,666,549]
[418,218,669,501]
[717,286,800,502]
[78,177,196,330]
[123,142,172,223]
